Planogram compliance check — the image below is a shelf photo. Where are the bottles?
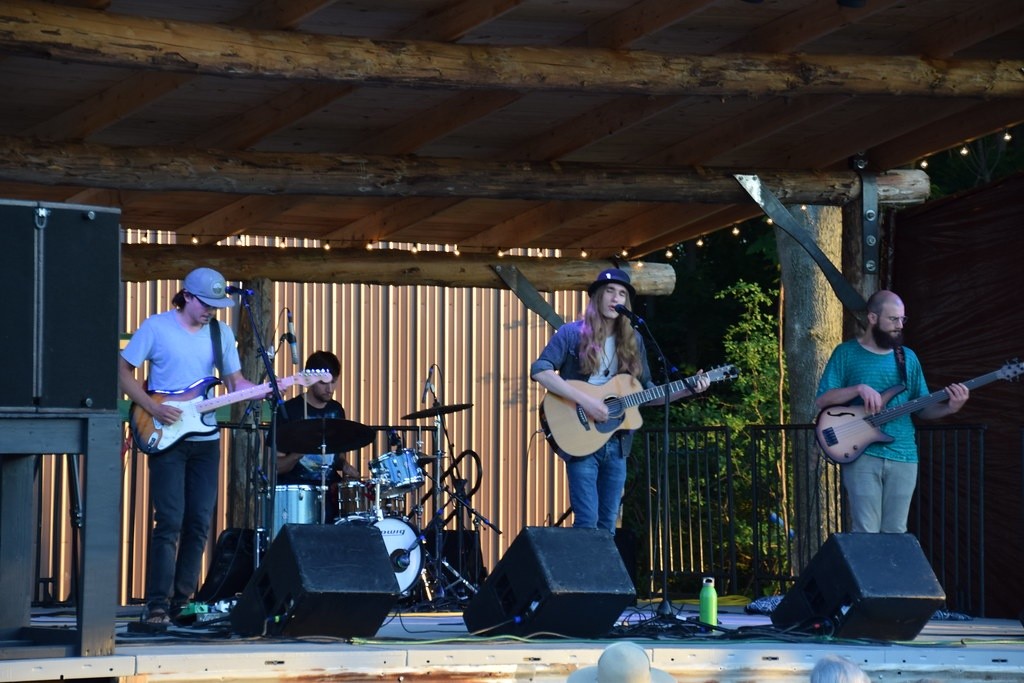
[699,577,717,632]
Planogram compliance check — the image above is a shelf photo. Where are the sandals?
[145,605,170,625]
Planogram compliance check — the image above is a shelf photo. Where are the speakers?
[769,532,947,642]
[226,522,401,642]
[464,526,637,640]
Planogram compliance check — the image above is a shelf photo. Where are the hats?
[588,269,636,298]
[183,268,236,307]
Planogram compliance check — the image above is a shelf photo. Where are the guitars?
[128,369,333,456]
[815,358,1024,464]
[538,363,737,463]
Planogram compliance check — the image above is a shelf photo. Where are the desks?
[0,406,122,658]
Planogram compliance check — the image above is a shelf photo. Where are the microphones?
[422,366,433,403]
[614,303,644,324]
[391,426,398,446]
[257,466,270,485]
[287,309,298,364]
[225,286,254,296]
[394,551,412,568]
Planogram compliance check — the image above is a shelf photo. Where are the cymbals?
[276,418,375,456]
[400,404,474,420]
[416,452,450,464]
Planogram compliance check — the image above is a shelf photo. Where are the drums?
[258,484,321,542]
[338,480,390,513]
[368,448,425,500]
[334,514,425,599]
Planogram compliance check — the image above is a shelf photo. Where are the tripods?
[619,323,742,637]
[404,413,479,616]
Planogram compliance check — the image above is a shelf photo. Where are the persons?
[267,352,359,523]
[530,268,712,537]
[121,267,290,628]
[815,289,970,533]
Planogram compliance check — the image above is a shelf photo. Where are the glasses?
[191,295,217,309]
